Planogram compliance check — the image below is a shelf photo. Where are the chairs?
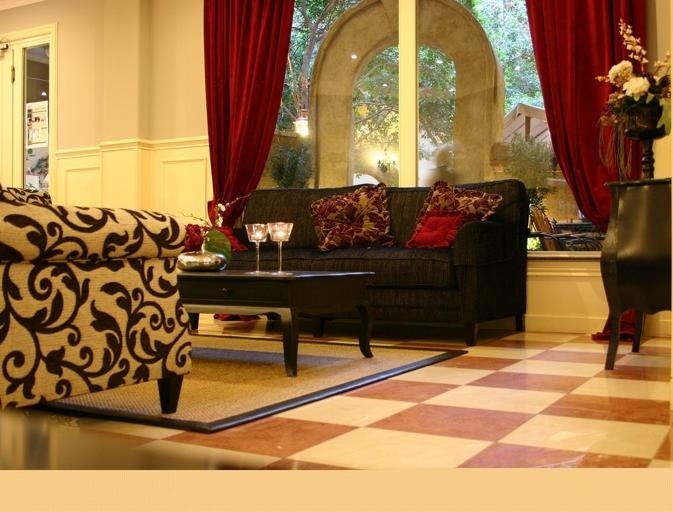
[529,204,598,252]
[599,180,669,371]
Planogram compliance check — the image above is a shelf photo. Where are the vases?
[177,238,228,273]
[624,108,668,179]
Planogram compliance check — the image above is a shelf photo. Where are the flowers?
[183,193,253,252]
[591,18,673,135]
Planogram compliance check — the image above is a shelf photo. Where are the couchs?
[0,183,187,415]
[231,177,531,346]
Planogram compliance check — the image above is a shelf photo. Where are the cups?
[244,224,270,274]
[267,222,293,277]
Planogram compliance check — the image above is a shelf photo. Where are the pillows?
[309,182,397,253]
[406,209,468,250]
[413,180,504,225]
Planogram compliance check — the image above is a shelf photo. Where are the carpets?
[23,346,468,434]
